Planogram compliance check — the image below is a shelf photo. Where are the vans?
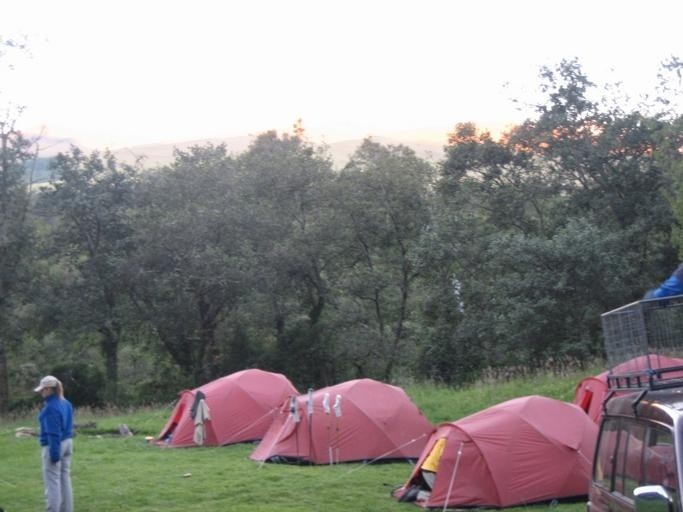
[586,388,683,511]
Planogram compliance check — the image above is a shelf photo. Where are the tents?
[602,430,677,492]
[390,393,599,512]
[574,353,683,430]
[147,368,301,448]
[249,377,435,465]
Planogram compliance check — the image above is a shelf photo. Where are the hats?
[33,376,59,393]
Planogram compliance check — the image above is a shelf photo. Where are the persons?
[34,375,74,512]
